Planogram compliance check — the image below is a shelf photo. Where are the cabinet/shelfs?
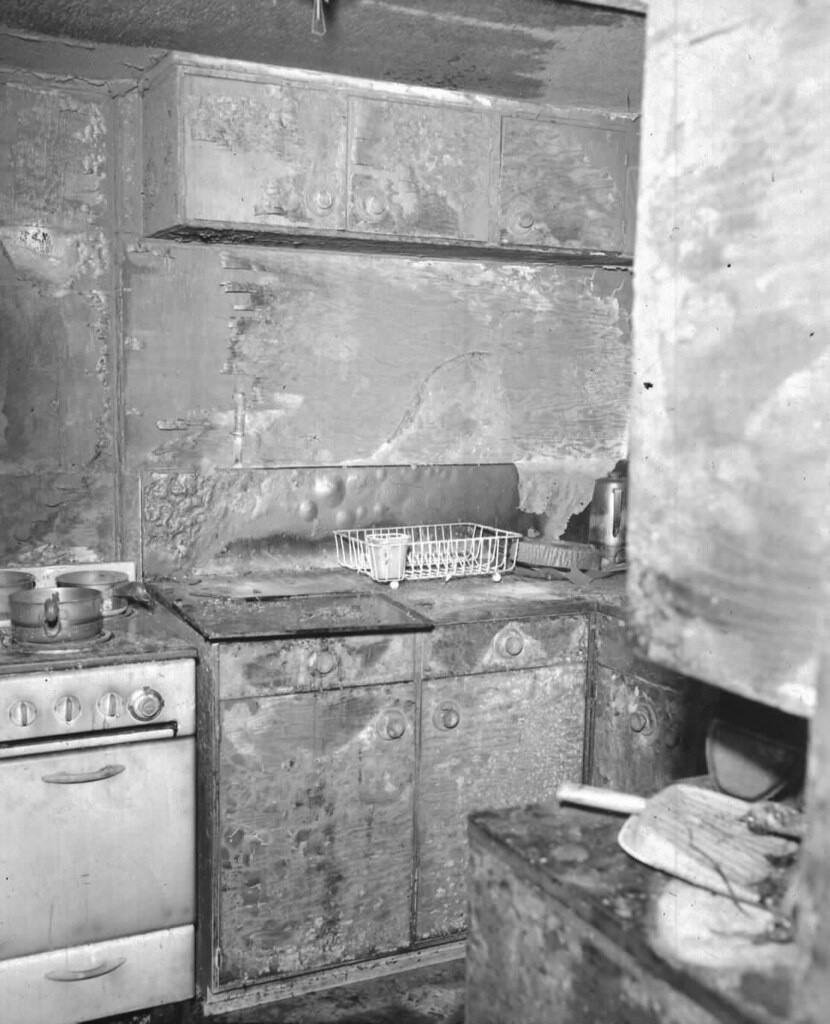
[583,584,807,805]
[140,51,642,265]
[148,562,589,1017]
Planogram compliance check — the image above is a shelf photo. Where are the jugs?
[588,472,626,563]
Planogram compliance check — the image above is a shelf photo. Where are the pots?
[55,569,151,617]
[0,570,35,616]
[8,587,104,643]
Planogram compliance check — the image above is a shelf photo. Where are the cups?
[368,533,411,580]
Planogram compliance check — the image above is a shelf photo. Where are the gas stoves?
[0,607,199,757]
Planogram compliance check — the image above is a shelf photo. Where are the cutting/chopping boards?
[555,780,796,907]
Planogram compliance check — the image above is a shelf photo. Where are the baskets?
[333,522,523,582]
